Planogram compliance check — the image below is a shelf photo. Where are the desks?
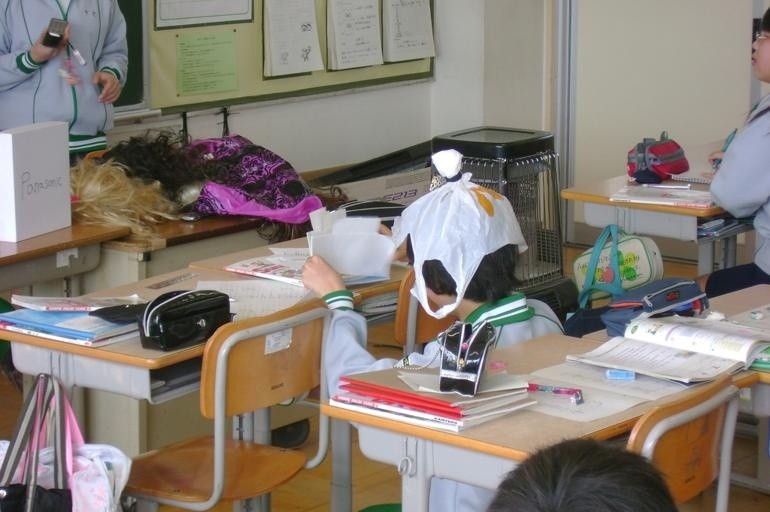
[2,138,769,512]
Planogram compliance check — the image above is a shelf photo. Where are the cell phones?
[46,16,65,41]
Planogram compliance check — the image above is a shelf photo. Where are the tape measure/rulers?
[146,271,200,290]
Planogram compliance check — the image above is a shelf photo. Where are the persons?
[483,438,681,512]
[300,151,567,512]
[691,6,770,298]
[0,1,130,165]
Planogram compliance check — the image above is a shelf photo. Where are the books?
[224,244,401,324]
[0,290,202,401]
[333,303,770,431]
[611,156,757,239]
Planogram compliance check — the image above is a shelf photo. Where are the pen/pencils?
[643,184,690,189]
[712,128,738,169]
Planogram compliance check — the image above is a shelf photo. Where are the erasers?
[751,312,763,318]
[605,369,635,380]
[42,19,69,49]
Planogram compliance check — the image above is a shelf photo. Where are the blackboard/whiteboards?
[112,0,150,113]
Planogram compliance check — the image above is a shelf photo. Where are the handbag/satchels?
[564,225,710,338]
[0,372,133,511]
